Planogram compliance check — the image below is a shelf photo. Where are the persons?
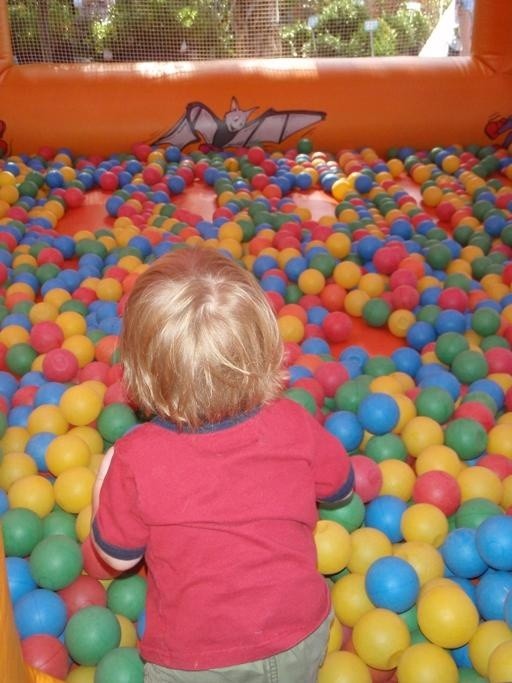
[89,243,355,683]
[6,0,473,63]
